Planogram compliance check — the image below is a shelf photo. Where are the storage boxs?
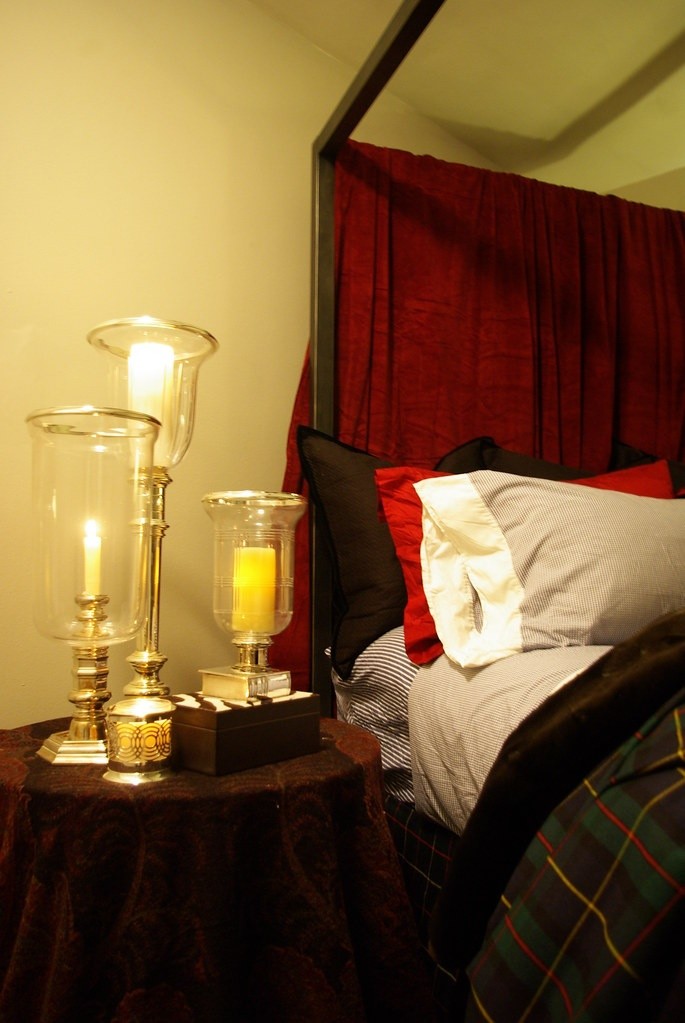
[158,690,321,778]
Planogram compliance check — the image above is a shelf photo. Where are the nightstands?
[0,709,401,1023]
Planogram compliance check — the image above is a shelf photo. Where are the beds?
[268,0,685,1023]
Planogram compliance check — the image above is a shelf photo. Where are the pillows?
[295,424,646,681]
[372,458,677,665]
[413,469,685,670]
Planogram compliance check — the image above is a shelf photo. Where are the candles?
[127,341,174,424]
[82,517,100,595]
[232,547,278,633]
